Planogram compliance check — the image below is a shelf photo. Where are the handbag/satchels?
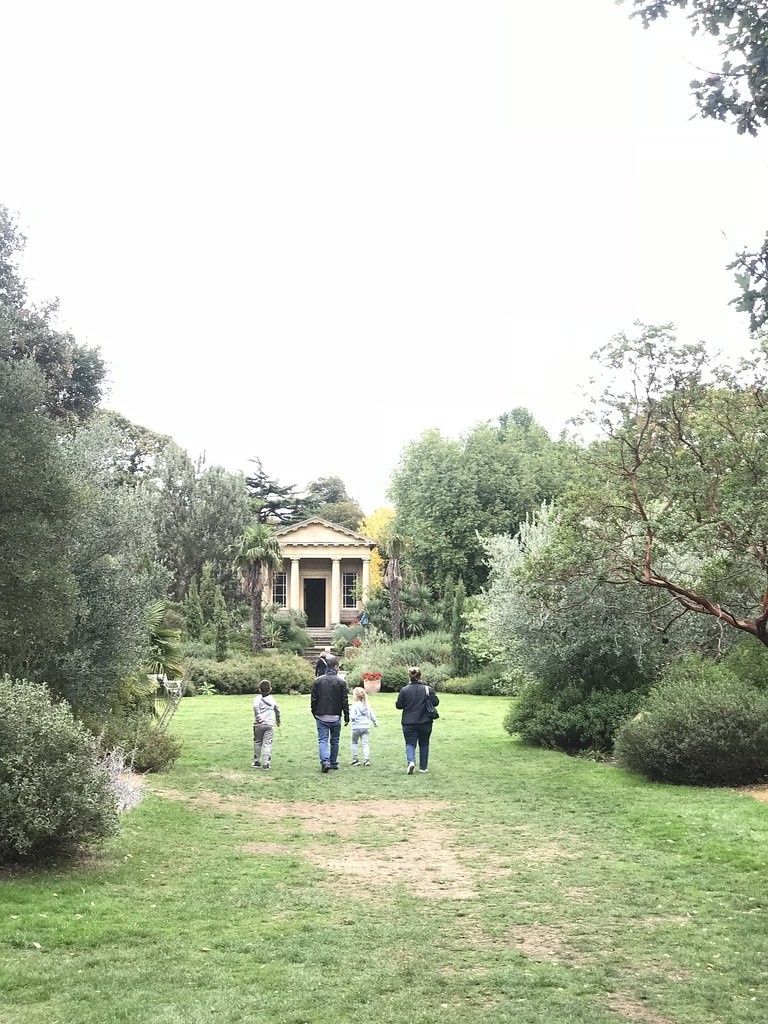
[424,684,439,720]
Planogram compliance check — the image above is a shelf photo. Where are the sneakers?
[407,762,415,774]
[350,759,361,766]
[363,759,370,765]
[419,768,429,772]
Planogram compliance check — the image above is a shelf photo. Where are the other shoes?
[321,765,330,773]
[262,764,271,770]
[329,764,339,769]
[250,761,261,768]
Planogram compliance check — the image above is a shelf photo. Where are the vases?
[363,679,382,694]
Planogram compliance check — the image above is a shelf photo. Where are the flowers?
[359,673,383,682]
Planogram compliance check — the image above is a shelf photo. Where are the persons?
[395,666,440,774]
[251,679,281,771]
[310,650,378,773]
